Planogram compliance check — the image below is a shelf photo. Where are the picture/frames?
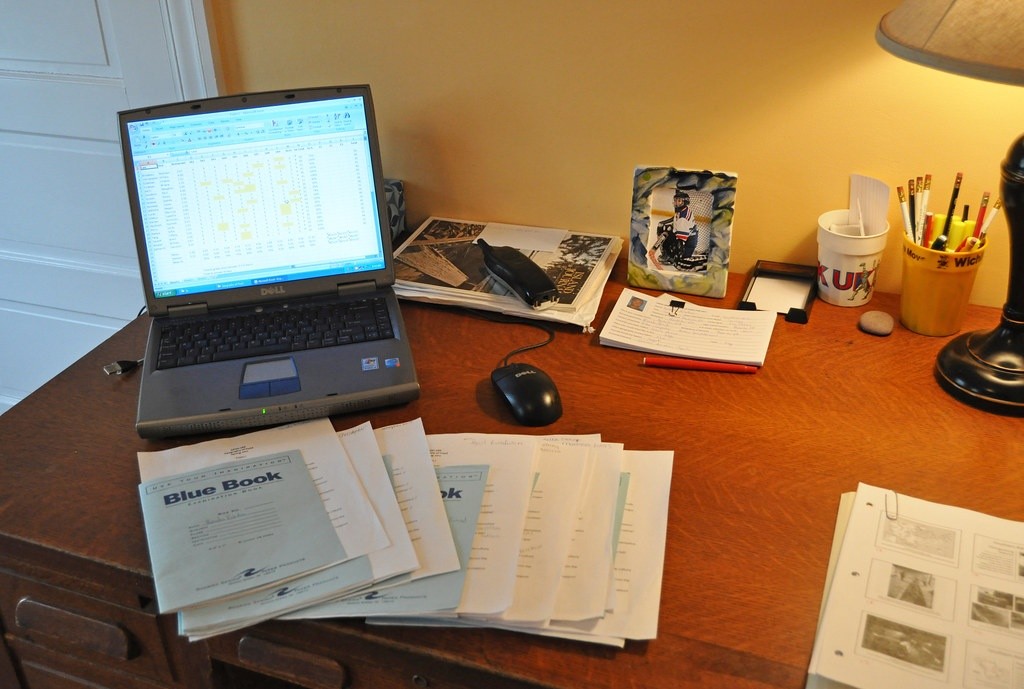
[627,165,738,298]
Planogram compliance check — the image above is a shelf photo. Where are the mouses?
[491,362,562,427]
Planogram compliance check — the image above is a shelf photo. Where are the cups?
[898,231,988,336]
[816,209,891,307]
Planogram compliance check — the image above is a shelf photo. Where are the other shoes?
[657,255,675,266]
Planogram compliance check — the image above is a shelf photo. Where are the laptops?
[114,83,420,439]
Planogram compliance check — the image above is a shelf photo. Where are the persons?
[655,191,709,273]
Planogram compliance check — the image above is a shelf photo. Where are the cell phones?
[477,238,560,311]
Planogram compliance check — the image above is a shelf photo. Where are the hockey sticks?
[648,232,669,270]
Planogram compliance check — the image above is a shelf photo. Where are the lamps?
[875,0,1024,418]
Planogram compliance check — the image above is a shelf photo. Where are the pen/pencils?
[893,165,1003,255]
[642,356,758,374]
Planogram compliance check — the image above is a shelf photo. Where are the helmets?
[674,192,690,213]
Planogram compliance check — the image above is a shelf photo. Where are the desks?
[0,256,1023,689]
